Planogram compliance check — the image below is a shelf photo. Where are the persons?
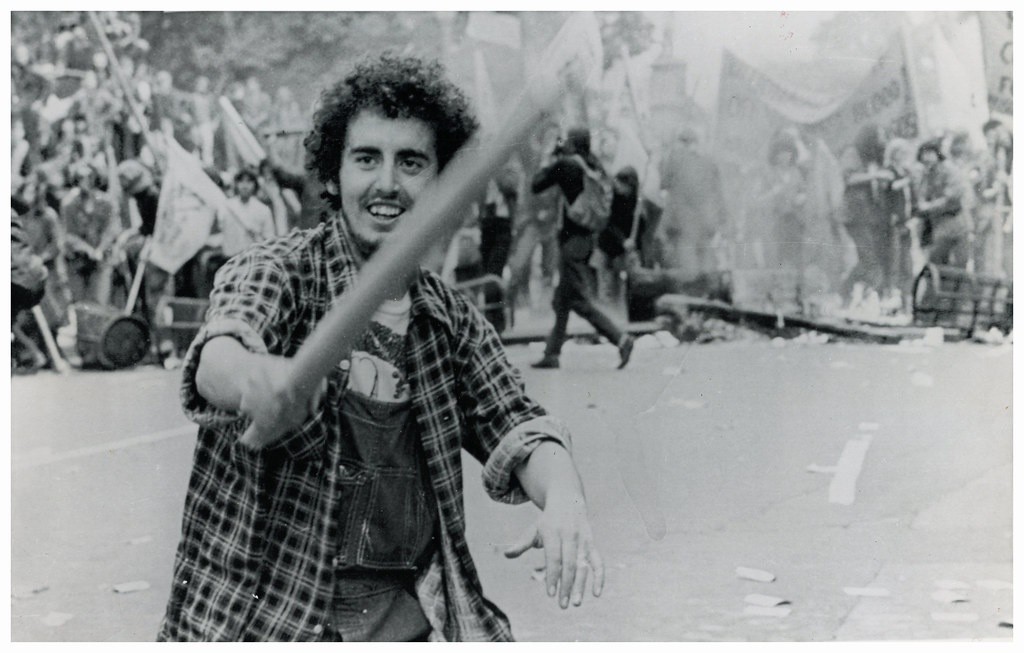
[219,167,275,260]
[11,10,1013,372]
[158,58,605,642]
[915,140,973,269]
[530,121,633,371]
[655,115,1014,328]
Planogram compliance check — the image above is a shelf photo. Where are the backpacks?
[562,155,614,231]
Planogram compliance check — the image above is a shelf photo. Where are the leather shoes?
[531,359,560,369]
[617,338,634,370]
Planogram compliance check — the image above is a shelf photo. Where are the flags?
[148,144,224,274]
[224,104,265,174]
